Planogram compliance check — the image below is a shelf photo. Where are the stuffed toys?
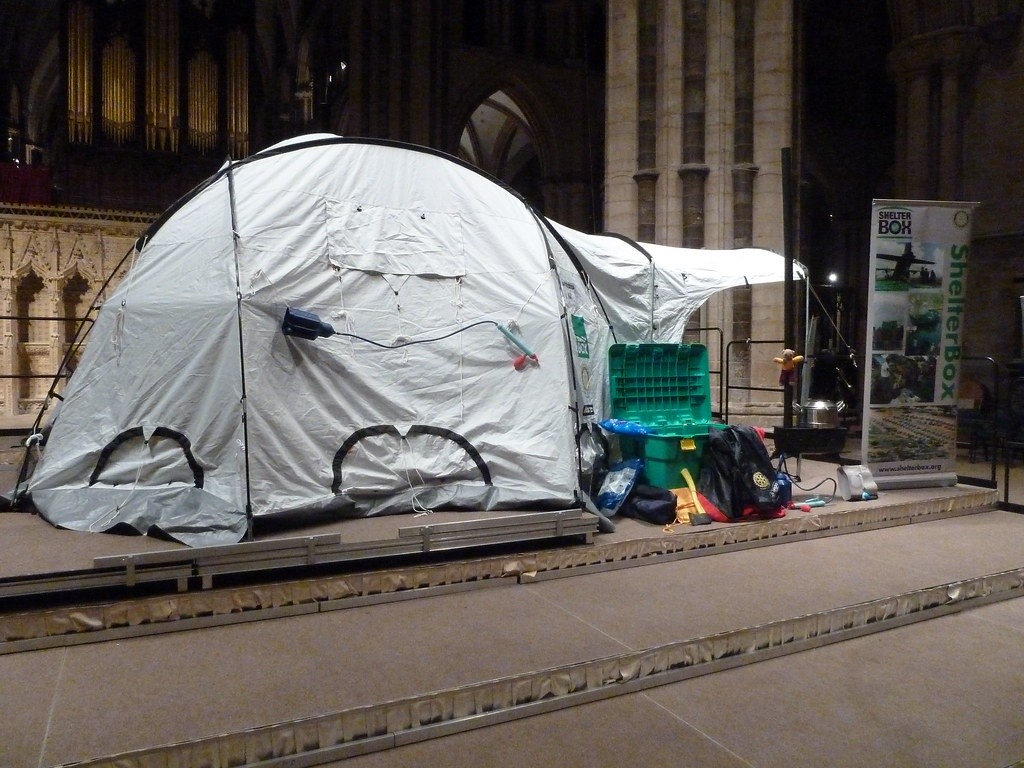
[771,348,805,387]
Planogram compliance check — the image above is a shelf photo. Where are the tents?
[0,132,811,554]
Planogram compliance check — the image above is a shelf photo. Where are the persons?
[919,266,937,286]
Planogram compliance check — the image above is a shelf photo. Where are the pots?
[791,399,846,429]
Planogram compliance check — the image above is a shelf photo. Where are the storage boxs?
[607,341,734,492]
